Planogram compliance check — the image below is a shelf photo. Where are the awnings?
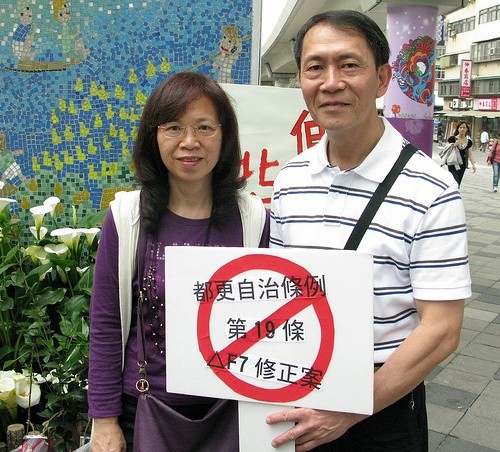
[433,110,500,119]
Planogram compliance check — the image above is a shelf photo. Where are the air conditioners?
[453,99,462,108]
[489,48,495,56]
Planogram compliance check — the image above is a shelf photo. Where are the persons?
[437,128,445,146]
[479,129,489,152]
[436,128,444,146]
[265,9,473,452]
[446,122,477,188]
[88,70,272,452]
[486,133,500,192]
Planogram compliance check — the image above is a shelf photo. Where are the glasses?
[157,121,222,139]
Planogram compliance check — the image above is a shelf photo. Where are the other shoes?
[493,187,497,192]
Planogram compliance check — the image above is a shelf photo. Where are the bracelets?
[473,162,476,164]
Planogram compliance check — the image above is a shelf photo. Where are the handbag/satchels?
[133,376,240,452]
[486,141,498,163]
